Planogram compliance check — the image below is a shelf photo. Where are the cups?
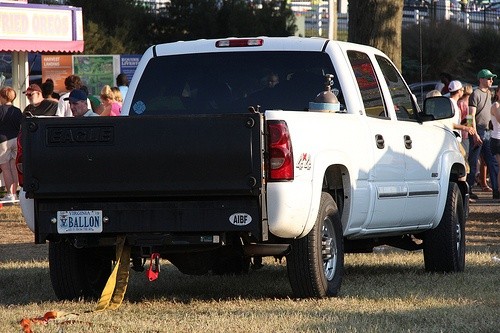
[467,115,473,128]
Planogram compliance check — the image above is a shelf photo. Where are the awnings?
[0,2,84,89]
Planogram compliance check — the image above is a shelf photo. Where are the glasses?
[485,78,493,81]
[100,95,107,100]
[26,93,35,96]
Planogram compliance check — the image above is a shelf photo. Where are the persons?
[426,69,500,202]
[0,73,130,203]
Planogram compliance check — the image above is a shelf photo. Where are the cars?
[407,76,499,101]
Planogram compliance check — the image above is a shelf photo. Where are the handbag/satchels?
[489,120,494,130]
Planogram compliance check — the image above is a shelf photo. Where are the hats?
[448,80,463,92]
[63,89,87,102]
[477,69,497,80]
[22,84,42,94]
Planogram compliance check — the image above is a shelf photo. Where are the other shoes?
[493,191,500,199]
[482,185,493,192]
[0,186,6,198]
[468,191,478,203]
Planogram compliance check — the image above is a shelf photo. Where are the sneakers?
[0,195,17,202]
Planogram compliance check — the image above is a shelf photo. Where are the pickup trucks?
[17,36,470,304]
